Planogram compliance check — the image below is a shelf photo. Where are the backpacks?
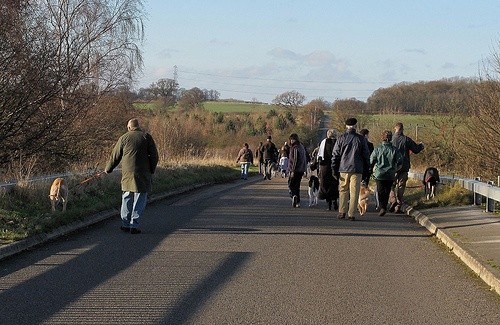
[242,149,250,159]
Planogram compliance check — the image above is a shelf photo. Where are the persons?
[106,119,160,235]
[287,132,306,208]
[333,117,368,221]
[254,135,288,181]
[236,143,255,180]
[312,123,423,217]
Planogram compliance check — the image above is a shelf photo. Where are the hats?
[267,135,271,139]
[345,118,357,126]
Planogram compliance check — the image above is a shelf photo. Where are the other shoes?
[129,228,141,234]
[292,195,297,208]
[333,200,339,210]
[395,210,400,213]
[347,216,356,221]
[379,208,387,216]
[325,208,331,210]
[119,226,130,232]
[263,175,266,180]
[388,202,397,213]
[337,213,346,219]
[295,203,301,208]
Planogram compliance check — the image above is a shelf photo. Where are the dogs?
[308,175,320,207]
[358,180,369,215]
[423,167,440,201]
[49,178,68,213]
